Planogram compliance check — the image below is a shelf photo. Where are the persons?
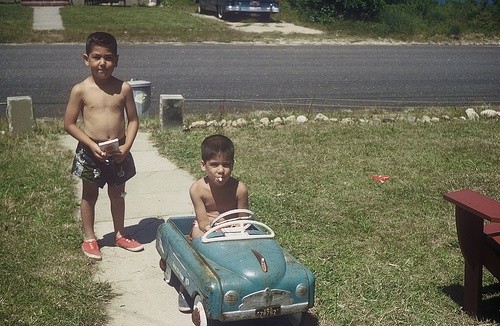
[65,32,144,259]
[189,134,248,240]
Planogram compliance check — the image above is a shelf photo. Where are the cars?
[156,209,316,326]
[195,0,280,20]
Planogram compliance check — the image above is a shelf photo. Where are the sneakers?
[114,234,144,251]
[81,240,101,260]
[178,290,194,312]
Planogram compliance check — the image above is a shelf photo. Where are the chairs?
[443,189,500,321]
